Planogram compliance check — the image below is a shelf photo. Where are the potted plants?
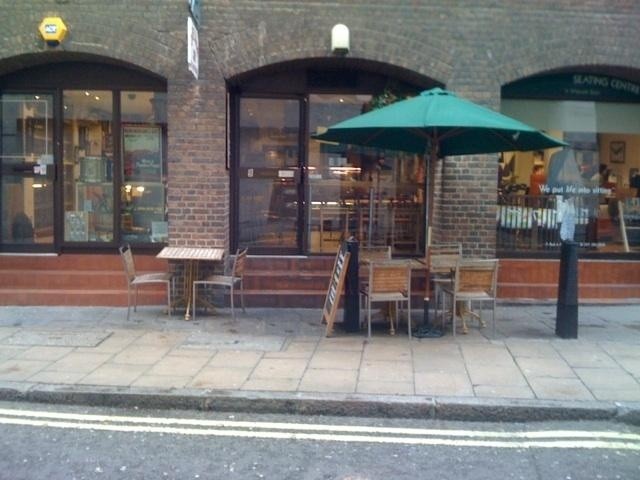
[609,141,626,163]
[563,131,599,151]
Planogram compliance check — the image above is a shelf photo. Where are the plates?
[75,182,165,242]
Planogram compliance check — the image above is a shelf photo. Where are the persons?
[590,163,609,188]
[529,165,547,196]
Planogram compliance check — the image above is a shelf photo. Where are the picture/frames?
[155,246,225,315]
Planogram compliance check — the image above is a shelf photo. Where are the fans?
[323,244,350,324]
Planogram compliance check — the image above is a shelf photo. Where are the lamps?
[192,245,250,322]
[118,242,176,319]
[319,207,421,254]
[358,240,502,340]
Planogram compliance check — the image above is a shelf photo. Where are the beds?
[310,86,571,328]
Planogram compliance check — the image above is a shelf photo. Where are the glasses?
[329,22,351,58]
